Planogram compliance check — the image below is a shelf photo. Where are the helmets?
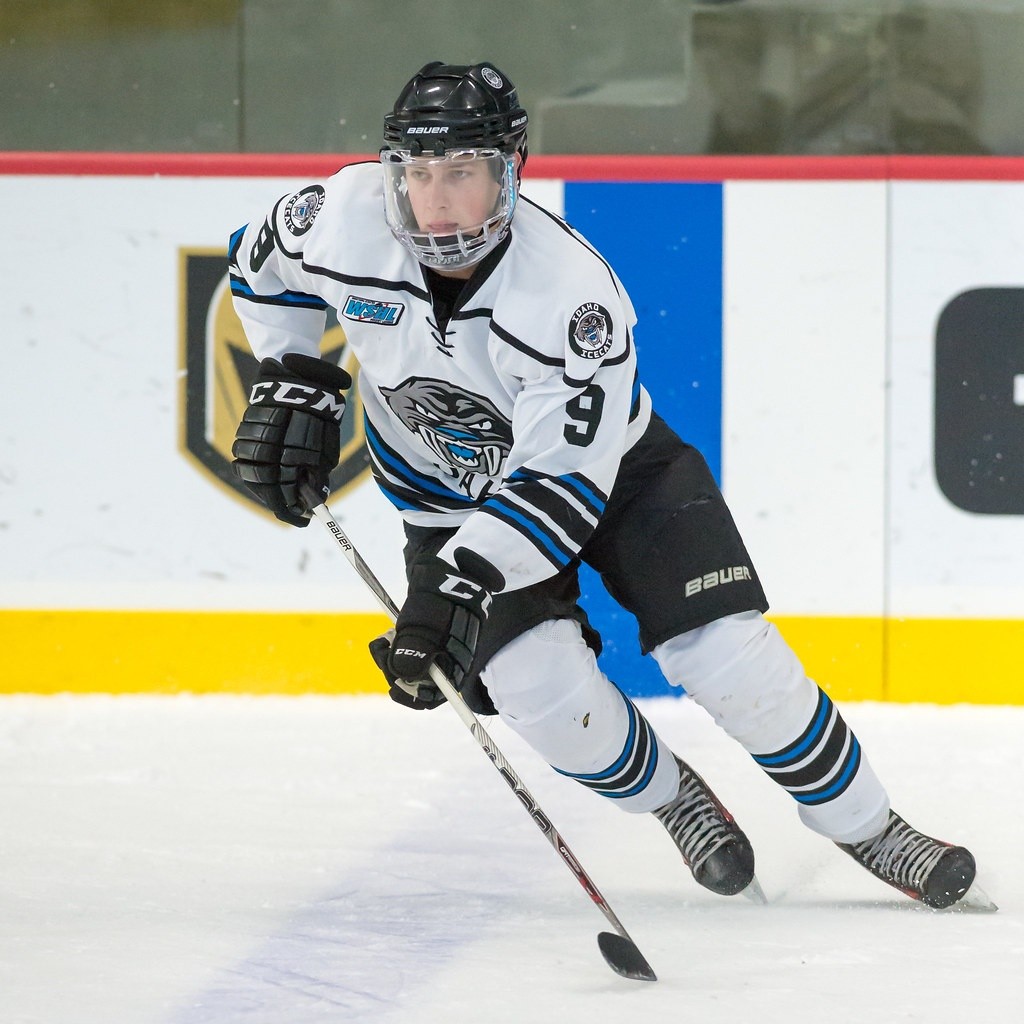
[365,59,530,270]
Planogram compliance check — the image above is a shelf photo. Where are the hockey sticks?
[292,474,666,989]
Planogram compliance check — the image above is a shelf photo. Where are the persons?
[228,61,999,914]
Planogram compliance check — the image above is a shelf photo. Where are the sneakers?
[649,751,767,904]
[831,806,998,913]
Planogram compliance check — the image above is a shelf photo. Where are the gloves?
[368,547,506,711]
[230,353,352,527]
